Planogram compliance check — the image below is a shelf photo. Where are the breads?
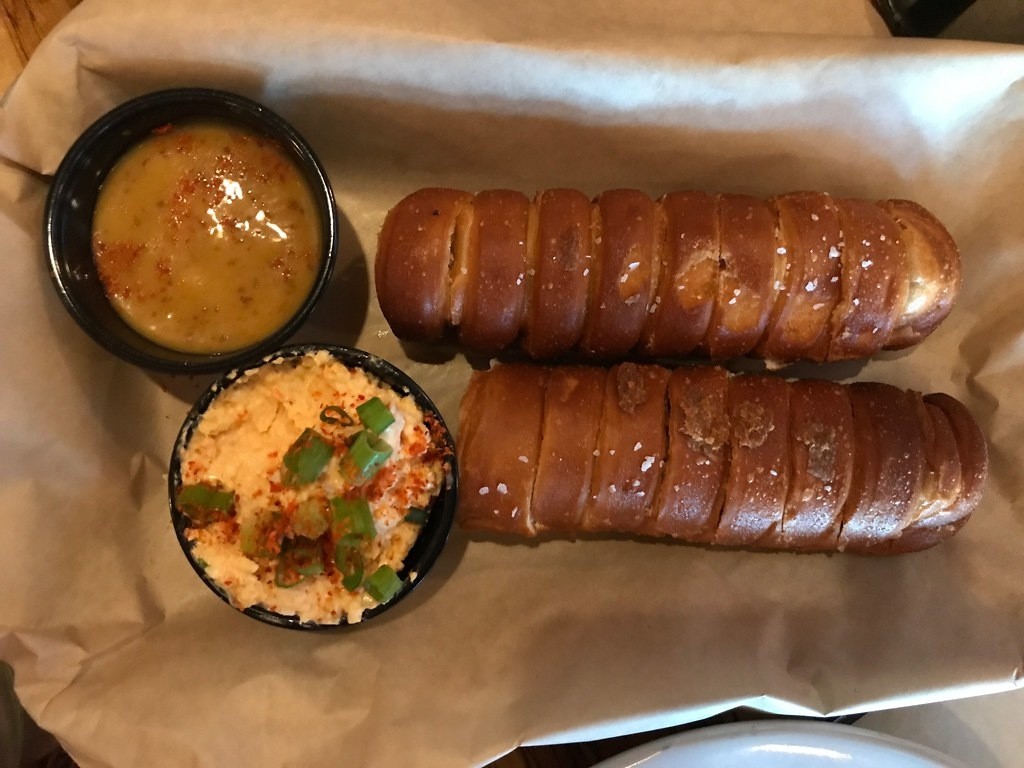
[451,361,989,557]
[374,177,961,371]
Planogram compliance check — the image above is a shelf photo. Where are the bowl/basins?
[42,86,336,373]
[166,341,461,632]
